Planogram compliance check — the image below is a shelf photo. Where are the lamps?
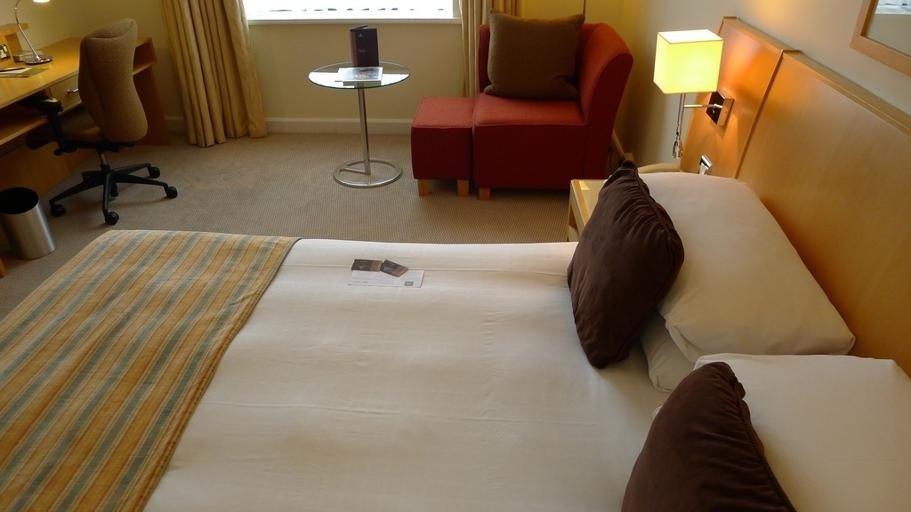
[651,29,736,161]
[13,1,54,66]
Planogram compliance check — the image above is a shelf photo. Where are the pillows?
[603,172,856,362]
[640,311,694,391]
[693,353,911,512]
[567,160,678,371]
[619,359,797,510]
[481,9,586,99]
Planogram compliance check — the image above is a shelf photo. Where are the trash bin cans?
[0,187,54,260]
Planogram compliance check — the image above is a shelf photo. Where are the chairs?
[472,20,635,199]
[47,18,182,225]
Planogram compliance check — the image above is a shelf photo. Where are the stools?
[409,95,479,196]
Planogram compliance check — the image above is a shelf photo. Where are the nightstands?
[565,179,608,242]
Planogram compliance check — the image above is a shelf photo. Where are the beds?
[0,16,911,510]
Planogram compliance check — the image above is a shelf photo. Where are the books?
[0,65,48,78]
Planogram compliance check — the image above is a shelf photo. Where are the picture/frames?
[849,1,911,79]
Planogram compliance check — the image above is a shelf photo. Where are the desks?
[1,22,170,278]
[308,61,413,189]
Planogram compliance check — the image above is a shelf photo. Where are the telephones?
[638,163,681,173]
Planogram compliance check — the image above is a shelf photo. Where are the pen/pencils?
[0,67,25,71]
